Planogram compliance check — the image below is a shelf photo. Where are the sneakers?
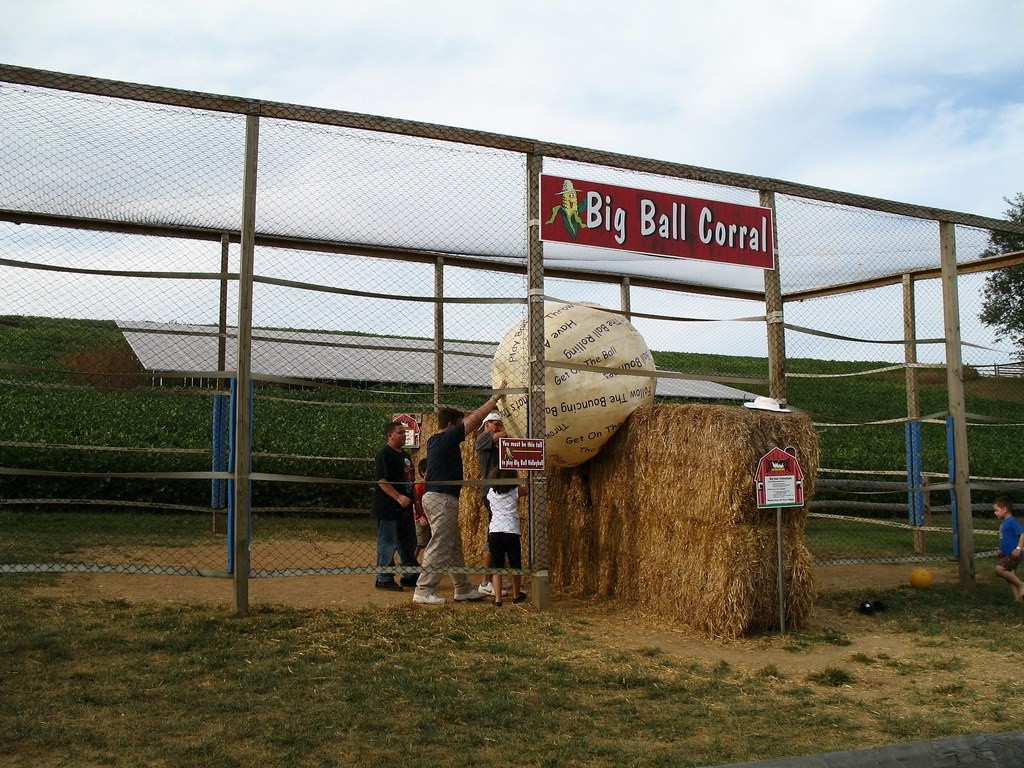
[478,582,508,596]
[501,581,512,588]
[454,589,486,601]
[413,593,446,604]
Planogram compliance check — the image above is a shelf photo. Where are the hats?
[477,412,502,431]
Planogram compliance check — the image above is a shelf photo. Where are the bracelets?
[491,397,497,402]
[1016,546,1021,551]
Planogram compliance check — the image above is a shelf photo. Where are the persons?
[413,380,507,604]
[485,466,531,608]
[370,422,421,591]
[413,457,432,558]
[475,413,508,595]
[993,495,1024,603]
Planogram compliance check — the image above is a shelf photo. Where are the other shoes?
[375,580,403,592]
[859,601,888,616]
[492,599,502,607]
[400,576,419,587]
[512,591,527,604]
